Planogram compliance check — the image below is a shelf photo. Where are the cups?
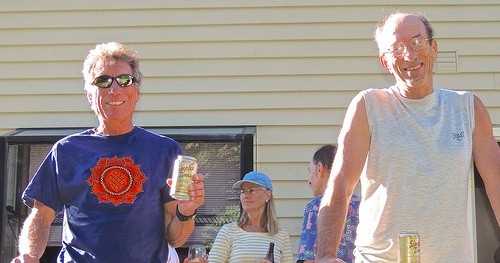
[188,244,206,263]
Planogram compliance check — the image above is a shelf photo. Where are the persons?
[208,171,296,263]
[167,242,208,263]
[297,144,362,263]
[10,42,205,263]
[315,12,500,263]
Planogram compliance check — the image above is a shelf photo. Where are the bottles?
[264,242,274,263]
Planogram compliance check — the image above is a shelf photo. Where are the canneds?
[169,154,198,200]
[398,232,421,263]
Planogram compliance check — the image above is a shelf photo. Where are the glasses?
[87,73,139,88]
[237,188,265,195]
[384,37,432,57]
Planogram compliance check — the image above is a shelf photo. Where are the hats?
[231,172,272,191]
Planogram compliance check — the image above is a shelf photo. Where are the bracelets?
[176,203,198,222]
